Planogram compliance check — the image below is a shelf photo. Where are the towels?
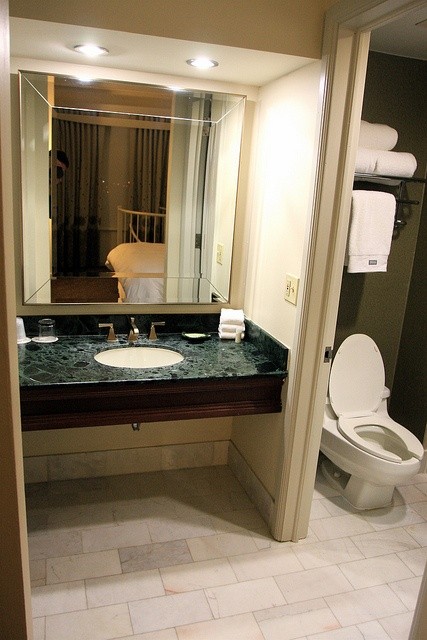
[357,121,399,151]
[343,191,395,275]
[352,147,418,179]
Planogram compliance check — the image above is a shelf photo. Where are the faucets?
[127,315,137,345]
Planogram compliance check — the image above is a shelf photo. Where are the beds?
[105,242,164,304]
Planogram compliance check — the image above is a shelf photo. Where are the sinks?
[92,343,185,369]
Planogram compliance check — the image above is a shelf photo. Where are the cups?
[37,319,57,341]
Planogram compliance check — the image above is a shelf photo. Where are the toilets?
[322,331,423,511]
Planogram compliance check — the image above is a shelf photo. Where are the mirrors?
[16,69,247,304]
